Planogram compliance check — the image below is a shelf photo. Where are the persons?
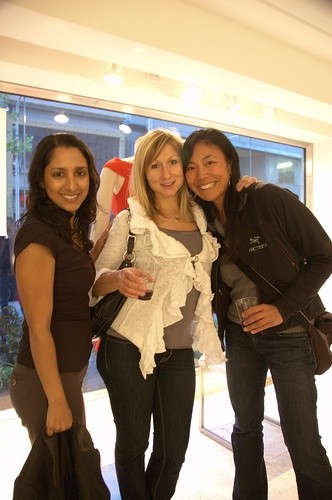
[183,129,332,500]
[88,129,228,500]
[8,133,113,452]
[90,155,135,249]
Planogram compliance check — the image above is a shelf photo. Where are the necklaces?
[162,212,179,222]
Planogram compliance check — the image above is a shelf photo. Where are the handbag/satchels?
[304,309,332,375]
[89,207,136,339]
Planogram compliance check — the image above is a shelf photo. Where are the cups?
[134,261,162,301]
[235,296,259,329]
